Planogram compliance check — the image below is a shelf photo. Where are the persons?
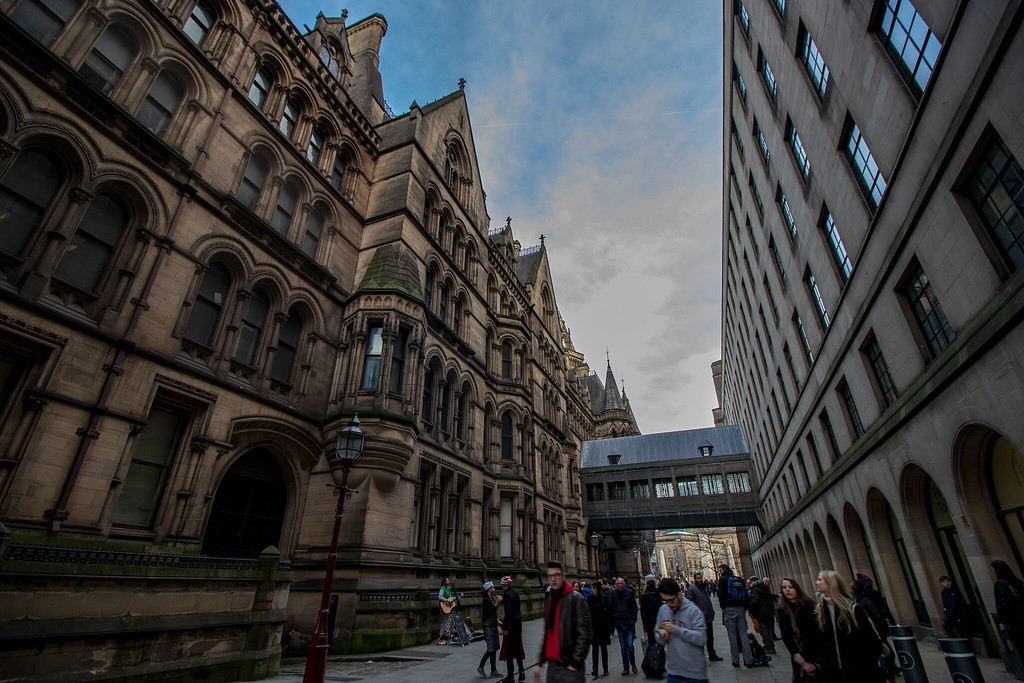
[654,579,709,683]
[676,575,719,597]
[940,576,971,638]
[533,560,638,683]
[717,564,779,668]
[775,570,902,683]
[477,576,526,683]
[438,577,464,641]
[991,560,1024,683]
[686,573,723,661]
[640,579,667,674]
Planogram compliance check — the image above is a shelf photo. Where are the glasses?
[547,573,563,580]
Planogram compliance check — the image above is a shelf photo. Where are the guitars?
[439,593,461,614]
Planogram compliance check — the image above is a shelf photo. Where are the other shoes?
[604,669,609,675]
[621,669,630,675]
[774,638,782,641]
[591,671,598,678]
[762,645,776,654]
[747,664,753,668]
[709,655,723,660]
[491,671,503,677]
[477,666,486,678]
[518,672,526,681]
[502,677,515,683]
[732,662,740,667]
[632,665,637,673]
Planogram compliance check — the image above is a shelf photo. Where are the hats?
[499,576,512,585]
[483,581,494,591]
[857,574,874,588]
[850,580,864,590]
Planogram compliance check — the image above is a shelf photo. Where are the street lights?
[300,413,366,683]
[632,549,641,604]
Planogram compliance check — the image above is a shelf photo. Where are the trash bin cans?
[991,612,1024,682]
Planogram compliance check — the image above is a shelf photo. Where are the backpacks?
[725,575,747,601]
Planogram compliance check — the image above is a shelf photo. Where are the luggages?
[642,645,666,679]
